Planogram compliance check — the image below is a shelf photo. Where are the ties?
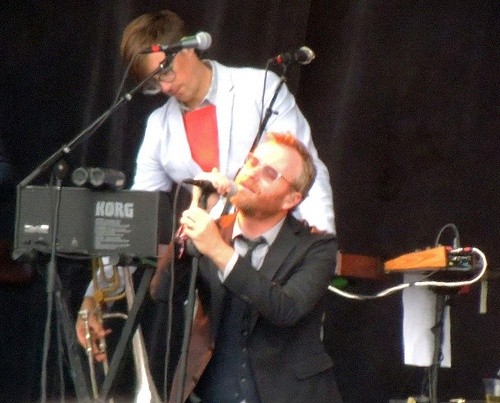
[235,235,266,264]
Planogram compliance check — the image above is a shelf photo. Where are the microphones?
[139,31,212,54]
[183,179,238,197]
[270,46,314,65]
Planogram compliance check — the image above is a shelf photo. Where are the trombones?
[78,255,163,403]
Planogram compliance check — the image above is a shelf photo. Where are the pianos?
[383,245,500,273]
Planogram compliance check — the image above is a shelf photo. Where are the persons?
[74,10,337,403]
[149,132,339,403]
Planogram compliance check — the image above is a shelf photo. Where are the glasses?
[138,68,177,96]
[242,153,297,193]
